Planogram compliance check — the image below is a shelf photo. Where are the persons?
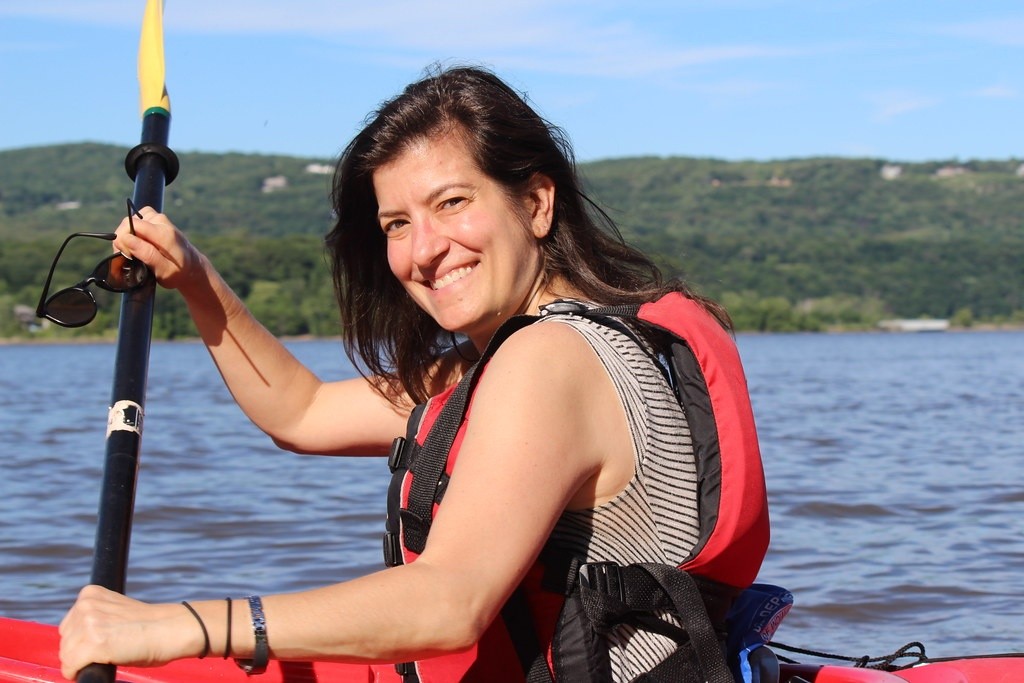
[56,66,729,683]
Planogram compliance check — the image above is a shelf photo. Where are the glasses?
[36,198,151,327]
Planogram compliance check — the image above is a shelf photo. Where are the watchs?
[233,595,269,675]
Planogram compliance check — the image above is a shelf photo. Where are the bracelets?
[223,597,232,660]
[181,600,210,659]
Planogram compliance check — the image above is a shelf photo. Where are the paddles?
[68,0,188,683]
